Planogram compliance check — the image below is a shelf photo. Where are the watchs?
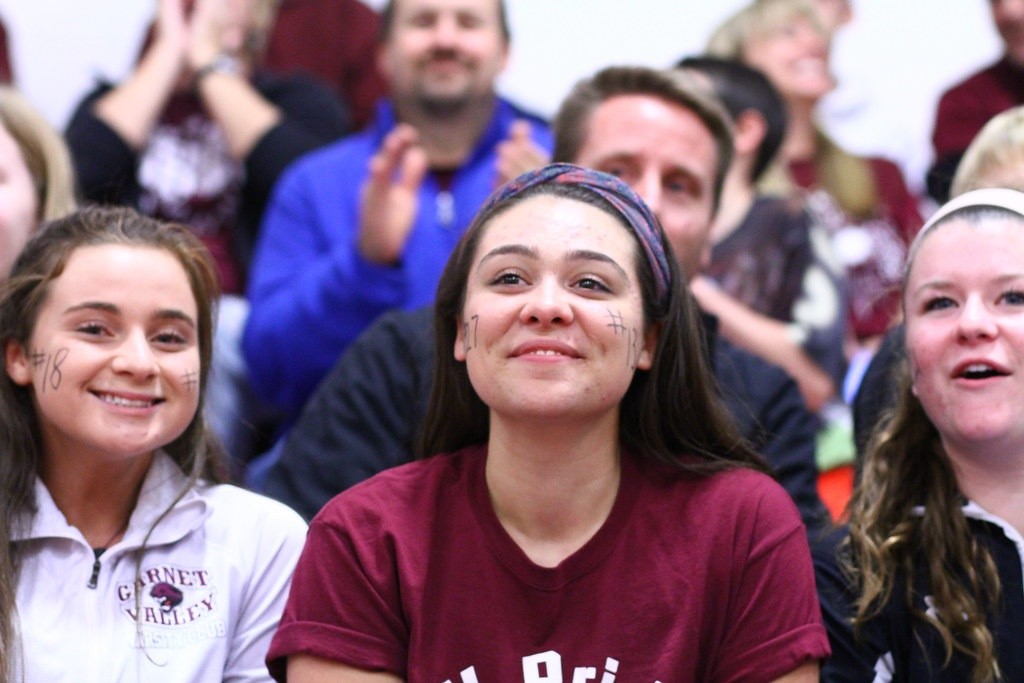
[192,55,236,84]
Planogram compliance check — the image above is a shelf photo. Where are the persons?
[0,0,1024,683]
